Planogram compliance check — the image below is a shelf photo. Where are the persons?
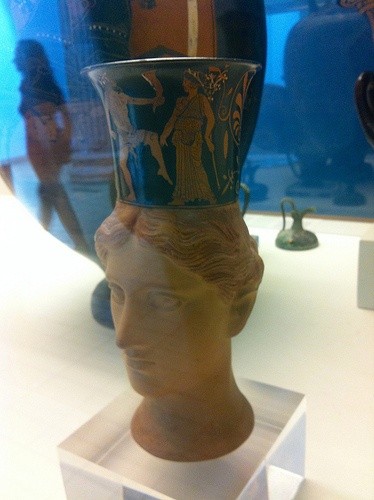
[11,40,95,258]
[93,201,264,462]
